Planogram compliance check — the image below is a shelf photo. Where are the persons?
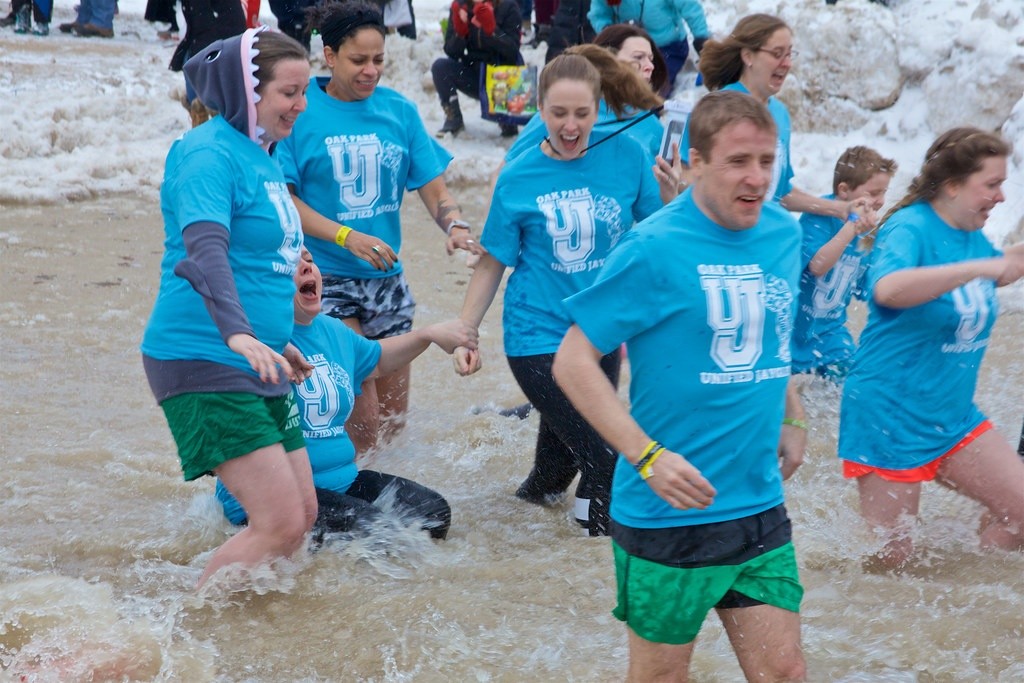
[491,25,669,257]
[783,147,897,393]
[216,242,480,565]
[837,127,1024,571]
[141,26,318,595]
[431,0,715,139]
[0,0,118,38]
[551,90,807,683]
[654,14,874,240]
[144,0,416,128]
[453,44,682,538]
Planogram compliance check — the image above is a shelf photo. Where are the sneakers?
[498,120,518,138]
[437,114,465,137]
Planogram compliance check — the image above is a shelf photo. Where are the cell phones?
[660,120,684,171]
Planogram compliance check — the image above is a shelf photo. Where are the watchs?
[447,219,471,236]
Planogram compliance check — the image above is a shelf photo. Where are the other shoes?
[157,29,180,41]
[34,21,50,36]
[71,22,114,38]
[13,3,31,33]
[59,21,79,32]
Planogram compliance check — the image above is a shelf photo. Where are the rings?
[466,240,474,244]
[666,177,671,185]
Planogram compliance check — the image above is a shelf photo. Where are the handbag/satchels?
[478,47,538,126]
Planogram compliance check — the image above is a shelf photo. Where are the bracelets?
[847,212,859,222]
[336,226,353,247]
[782,418,807,431]
[633,440,666,473]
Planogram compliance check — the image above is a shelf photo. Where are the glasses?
[752,47,799,62]
